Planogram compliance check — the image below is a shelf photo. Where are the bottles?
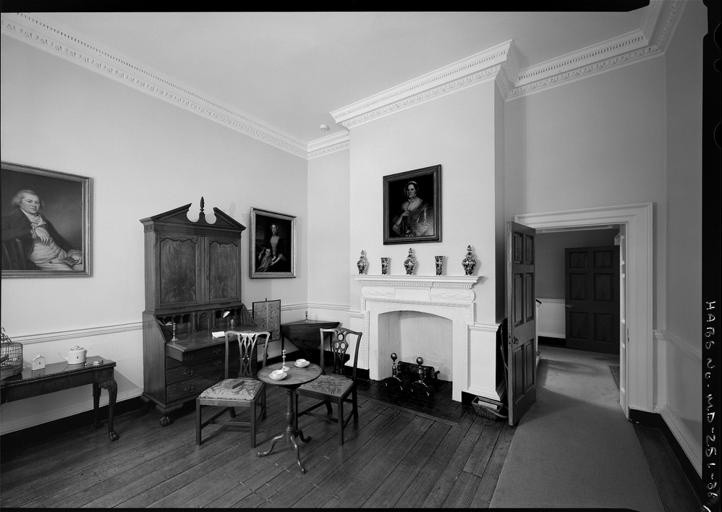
[435,255,445,276]
[402,247,416,275]
[356,250,369,277]
[381,256,391,275]
[460,245,476,277]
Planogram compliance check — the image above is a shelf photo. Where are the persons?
[0,188,84,271]
[257,223,290,272]
[393,181,434,236]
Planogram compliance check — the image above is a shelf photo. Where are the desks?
[0,356,120,442]
[256,361,324,476]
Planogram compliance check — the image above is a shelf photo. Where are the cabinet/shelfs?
[142,304,261,427]
[139,194,248,313]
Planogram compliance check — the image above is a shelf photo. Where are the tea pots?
[59,345,88,365]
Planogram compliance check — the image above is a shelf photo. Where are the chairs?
[194,330,271,449]
[293,326,363,445]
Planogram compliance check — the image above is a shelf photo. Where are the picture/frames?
[249,206,297,279]
[0,161,94,278]
[382,164,443,245]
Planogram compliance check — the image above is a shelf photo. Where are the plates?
[268,359,312,381]
